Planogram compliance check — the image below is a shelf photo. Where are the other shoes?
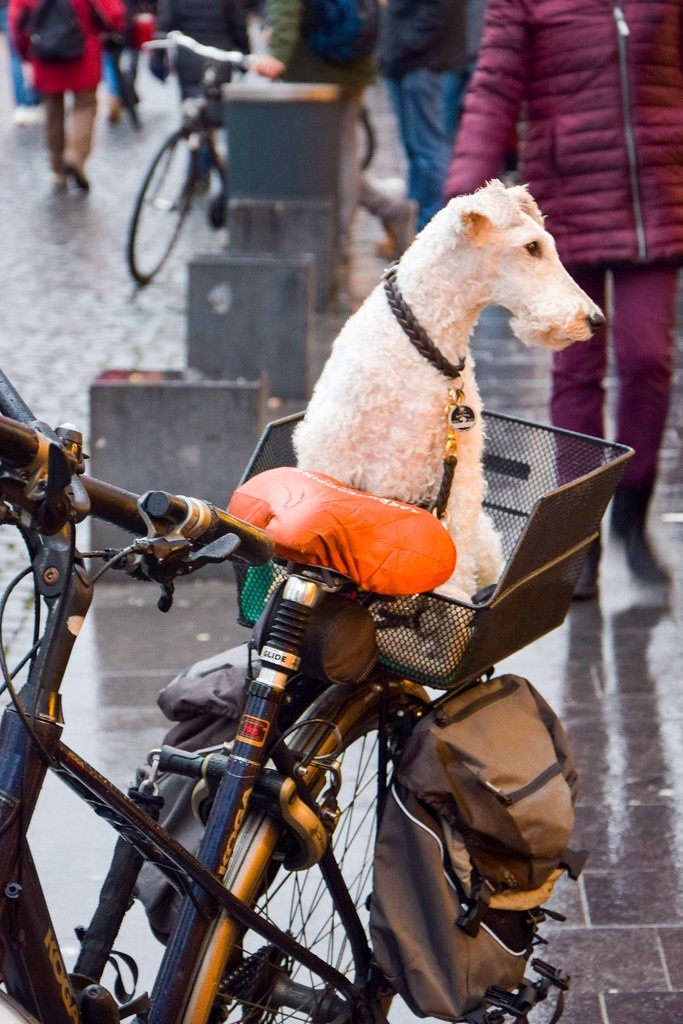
[383,201,419,254]
[51,172,67,190]
[14,103,47,127]
[109,104,119,123]
[61,150,89,190]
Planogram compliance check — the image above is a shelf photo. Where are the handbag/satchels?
[369,673,589,1024]
[131,640,262,947]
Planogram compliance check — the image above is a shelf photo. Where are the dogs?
[262,173,610,681]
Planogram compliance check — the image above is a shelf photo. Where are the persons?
[443,0,683,600]
[0,0,523,262]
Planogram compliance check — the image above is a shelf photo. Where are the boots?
[573,528,602,600]
[611,482,671,587]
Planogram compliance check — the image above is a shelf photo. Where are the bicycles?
[124,31,378,286]
[0,366,636,1024]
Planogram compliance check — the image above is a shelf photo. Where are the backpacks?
[28,0,83,63]
[302,0,380,66]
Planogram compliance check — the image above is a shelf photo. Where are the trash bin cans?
[225,81,350,203]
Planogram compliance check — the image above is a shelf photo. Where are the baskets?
[232,409,636,691]
